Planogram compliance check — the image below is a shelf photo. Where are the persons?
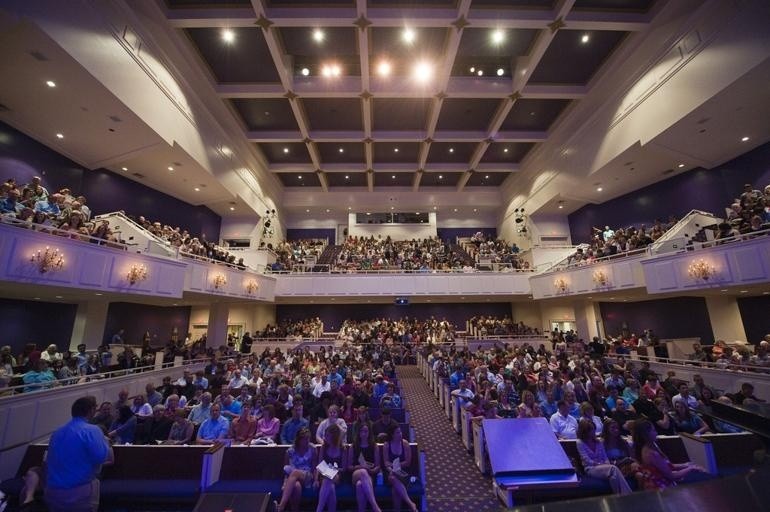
[184,385,202,414]
[91,421,115,480]
[335,235,464,273]
[639,360,658,386]
[91,402,113,433]
[516,389,536,419]
[605,385,636,416]
[549,327,661,362]
[195,403,229,445]
[187,393,213,427]
[634,389,658,420]
[282,395,311,421]
[478,381,498,400]
[470,232,534,272]
[735,384,758,404]
[119,210,244,269]
[384,425,419,512]
[316,404,345,444]
[312,392,332,424]
[131,394,153,418]
[43,395,112,511]
[655,388,669,407]
[280,404,310,445]
[642,374,663,403]
[327,379,342,412]
[470,313,541,339]
[725,393,742,405]
[569,216,678,268]
[536,381,548,403]
[272,239,323,274]
[672,398,711,436]
[235,385,252,406]
[589,391,612,422]
[213,384,235,408]
[140,333,415,387]
[577,421,633,494]
[255,403,280,444]
[217,393,242,421]
[227,403,257,445]
[632,417,706,490]
[503,374,522,406]
[531,402,543,417]
[373,406,401,442]
[258,382,268,401]
[565,389,581,424]
[253,317,322,340]
[340,396,357,423]
[540,389,559,422]
[714,184,770,245]
[691,375,715,401]
[666,376,680,408]
[278,385,293,410]
[352,406,369,442]
[450,379,474,410]
[465,394,485,415]
[602,418,642,478]
[272,429,317,512]
[660,370,676,389]
[648,398,675,437]
[248,394,263,420]
[105,405,137,445]
[613,398,635,436]
[346,421,382,512]
[380,382,401,407]
[112,388,134,409]
[145,383,165,407]
[1,177,117,246]
[549,400,579,466]
[267,390,288,424]
[164,407,195,446]
[497,390,515,418]
[163,394,180,417]
[164,385,187,411]
[671,383,704,418]
[3,466,43,512]
[352,380,371,409]
[579,401,603,437]
[712,396,744,434]
[141,403,172,445]
[0,329,140,394]
[468,400,505,456]
[336,316,457,343]
[420,342,549,383]
[315,424,346,512]
[300,382,319,417]
[685,334,770,373]
[549,362,639,394]
[698,387,716,407]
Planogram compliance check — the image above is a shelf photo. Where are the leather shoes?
[273,500,278,512]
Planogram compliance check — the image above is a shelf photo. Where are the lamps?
[687,257,715,282]
[209,274,227,291]
[244,279,258,296]
[594,269,609,288]
[125,264,146,284]
[553,276,570,294]
[30,244,61,275]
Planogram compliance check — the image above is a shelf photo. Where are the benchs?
[493,437,635,509]
[295,237,326,270]
[15,443,212,505]
[213,443,427,511]
[459,235,498,264]
